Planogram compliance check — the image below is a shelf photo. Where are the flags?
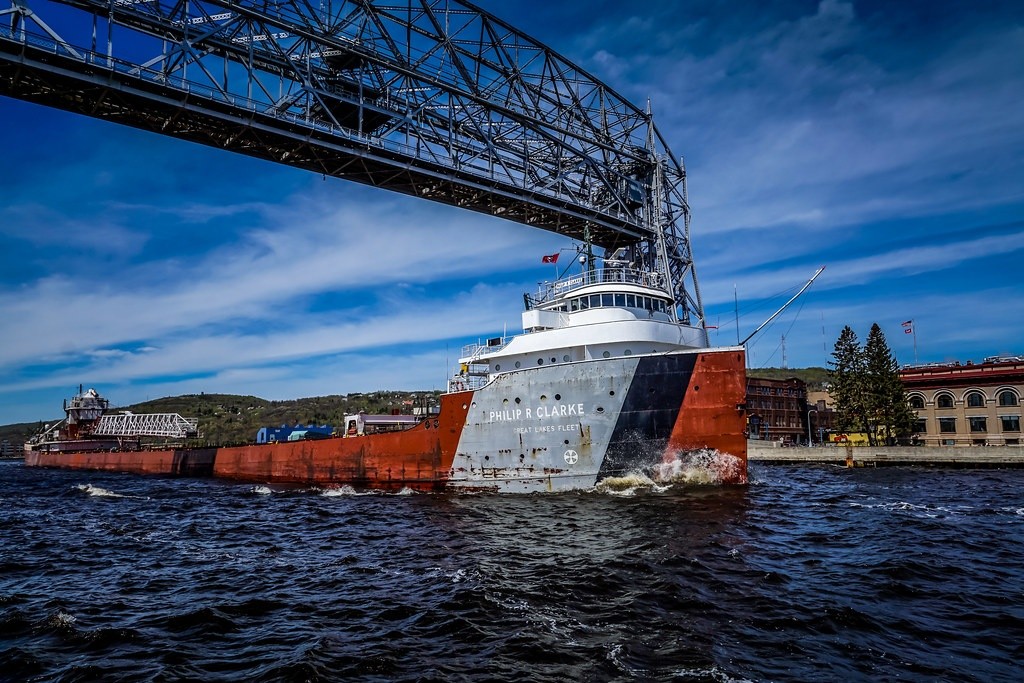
[902,320,911,327]
[905,328,912,333]
[542,253,559,264]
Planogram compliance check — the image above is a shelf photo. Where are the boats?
[18,222,751,497]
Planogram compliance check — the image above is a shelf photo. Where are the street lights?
[807,409,817,447]
